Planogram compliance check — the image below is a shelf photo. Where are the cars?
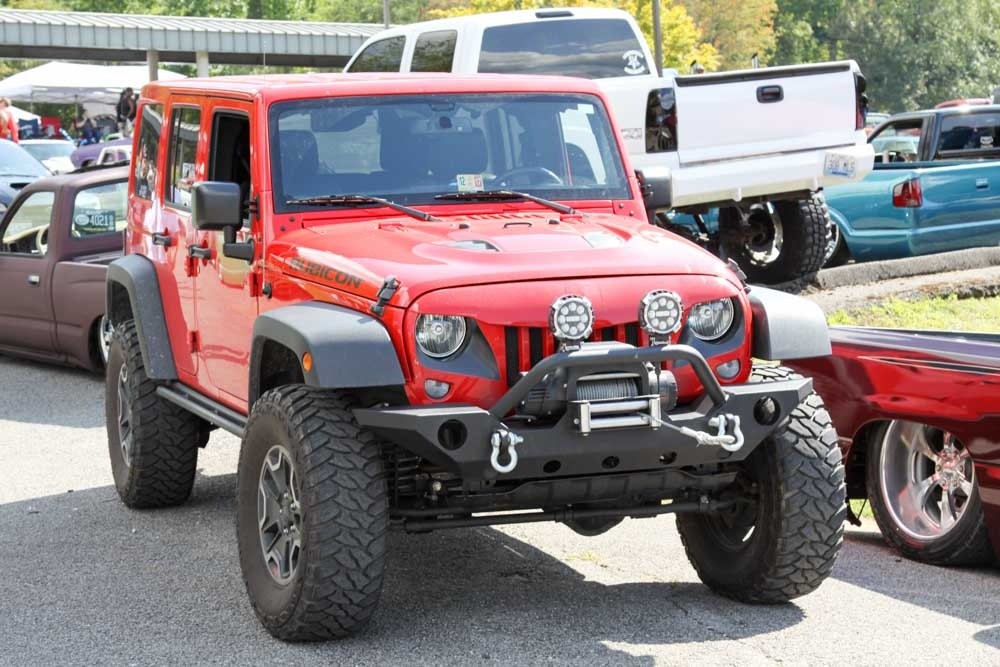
[0,138,132,372]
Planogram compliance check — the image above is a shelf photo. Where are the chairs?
[235,130,319,205]
[408,124,506,189]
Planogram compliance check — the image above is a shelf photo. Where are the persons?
[75,109,100,143]
[116,88,136,137]
[0,99,18,143]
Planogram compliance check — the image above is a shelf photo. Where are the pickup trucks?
[866,98,1000,162]
[780,326,1000,567]
[340,7,875,283]
[657,161,1000,268]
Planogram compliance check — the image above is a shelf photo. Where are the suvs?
[104,74,848,642]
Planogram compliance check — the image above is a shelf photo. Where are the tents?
[1,62,187,105]
